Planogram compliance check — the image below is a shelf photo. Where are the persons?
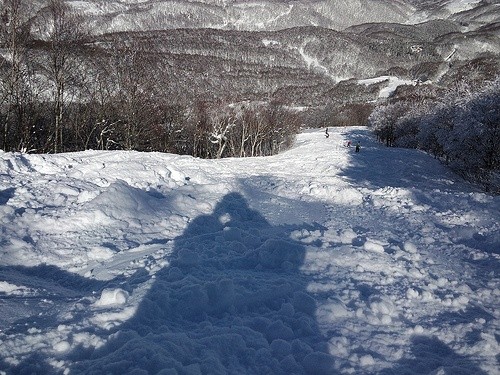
[354,140,361,152]
[347,140,351,147]
